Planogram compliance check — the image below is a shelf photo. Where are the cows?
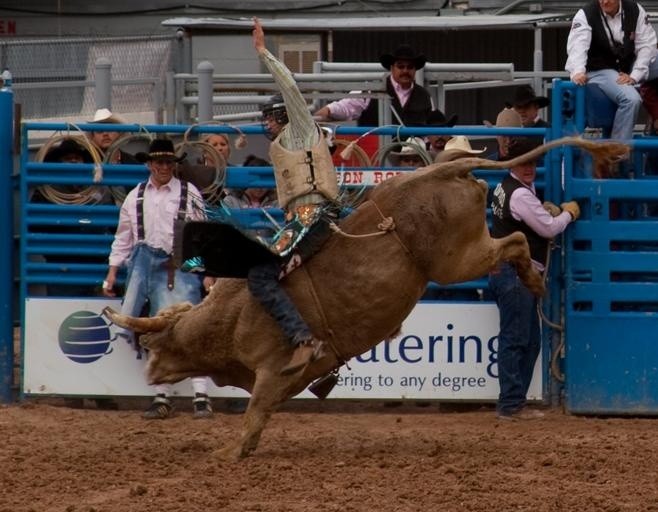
[101,135,632,462]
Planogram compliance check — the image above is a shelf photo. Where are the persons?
[86,46,658,305]
[564,1,658,177]
[494,144,582,425]
[30,139,121,411]
[246,17,344,377]
[101,139,217,422]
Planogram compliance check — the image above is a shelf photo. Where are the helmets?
[261,92,290,141]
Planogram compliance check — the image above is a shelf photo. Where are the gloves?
[561,200,581,221]
[542,201,560,217]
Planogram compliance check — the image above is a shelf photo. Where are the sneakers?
[278,337,324,376]
[191,397,214,420]
[500,408,545,421]
[140,397,173,418]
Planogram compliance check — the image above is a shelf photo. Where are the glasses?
[396,61,415,70]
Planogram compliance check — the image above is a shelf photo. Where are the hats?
[389,85,551,163]
[86,108,124,123]
[380,38,426,71]
[57,139,84,156]
[135,139,190,164]
